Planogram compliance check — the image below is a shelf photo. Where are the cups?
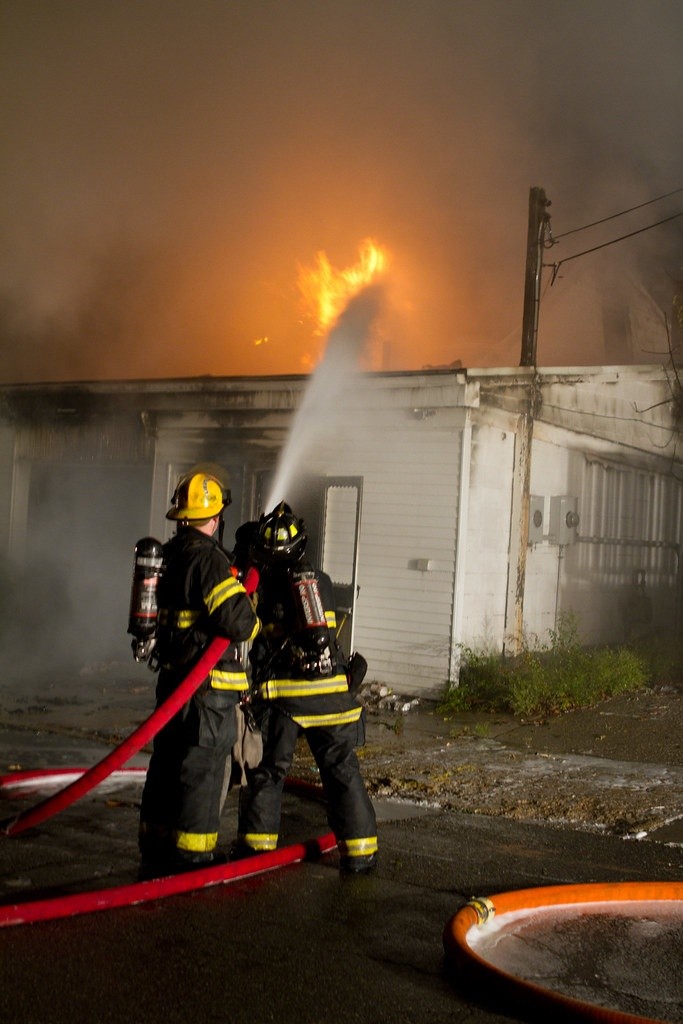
[370,684,388,697]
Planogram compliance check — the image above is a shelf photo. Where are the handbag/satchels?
[233,703,264,768]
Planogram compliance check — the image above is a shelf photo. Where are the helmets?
[255,501,308,562]
[166,474,231,522]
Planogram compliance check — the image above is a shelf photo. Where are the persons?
[232,505,378,871]
[137,471,261,884]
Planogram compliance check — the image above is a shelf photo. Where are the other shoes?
[342,852,378,872]
[176,850,225,872]
[138,853,172,878]
[231,839,268,858]
